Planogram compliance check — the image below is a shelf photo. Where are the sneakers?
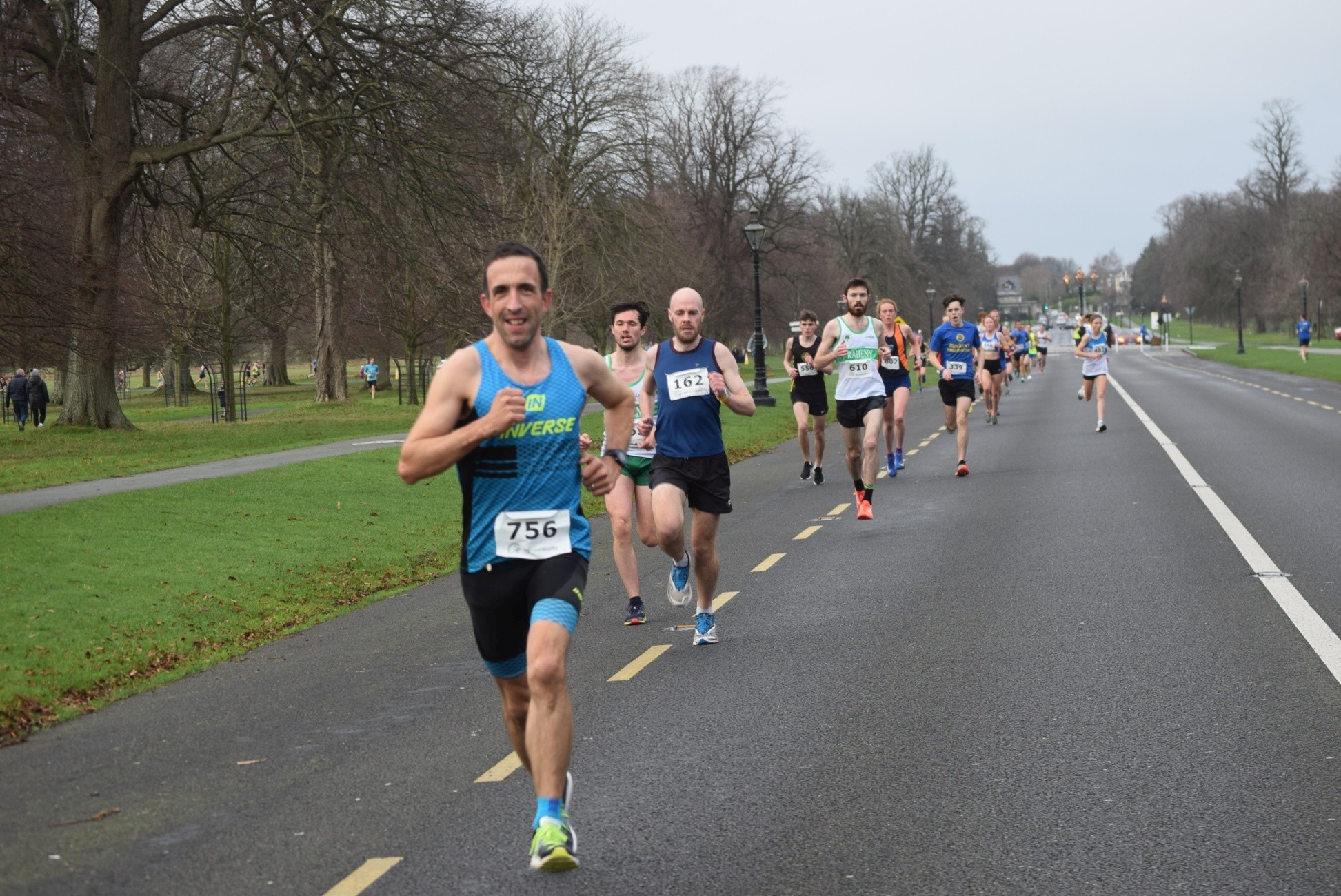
[917,362,1046,401]
[1095,421,1106,432]
[623,602,647,625]
[946,421,955,435]
[800,460,815,481]
[528,815,580,876]
[812,465,825,485]
[985,412,998,425]
[886,452,898,478]
[895,449,905,471]
[693,608,721,646]
[853,478,867,514]
[555,767,573,822]
[955,463,969,477]
[856,498,875,522]
[666,550,692,608]
[1077,384,1086,400]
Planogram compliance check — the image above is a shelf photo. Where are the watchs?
[603,448,626,468]
[719,389,730,404]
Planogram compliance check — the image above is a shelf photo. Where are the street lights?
[1298,274,1311,346]
[1317,297,1324,341]
[1062,268,1099,318]
[1233,269,1247,354]
[925,280,935,342]
[742,202,780,407]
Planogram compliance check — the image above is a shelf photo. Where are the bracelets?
[940,367,945,373]
[916,356,921,359]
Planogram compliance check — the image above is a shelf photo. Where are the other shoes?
[967,404,973,413]
[18,421,24,432]
[37,423,43,429]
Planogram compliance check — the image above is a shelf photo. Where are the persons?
[637,286,755,647]
[875,298,922,477]
[1294,314,1312,363]
[927,292,985,476]
[577,300,660,627]
[0,356,381,436]
[909,334,930,393]
[970,307,1150,433]
[392,242,638,878]
[782,308,832,485]
[812,278,892,519]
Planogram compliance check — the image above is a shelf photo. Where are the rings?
[591,483,595,491]
